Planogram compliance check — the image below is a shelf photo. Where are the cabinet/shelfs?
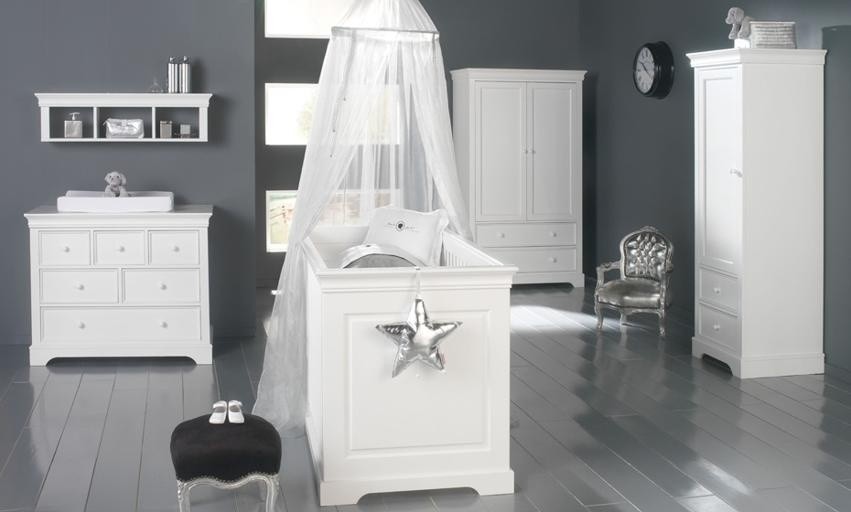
[33,90,213,143]
[685,48,829,378]
[455,66,586,289]
[23,199,217,368]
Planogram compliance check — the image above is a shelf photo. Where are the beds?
[304,224,518,505]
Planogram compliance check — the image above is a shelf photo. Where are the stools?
[169,412,283,512]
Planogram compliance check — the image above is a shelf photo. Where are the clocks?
[631,41,674,100]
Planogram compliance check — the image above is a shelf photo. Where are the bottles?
[178,55,191,93]
[166,56,178,93]
[159,120,172,138]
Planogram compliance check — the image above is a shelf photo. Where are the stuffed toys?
[725,7,753,39]
[104,171,128,197]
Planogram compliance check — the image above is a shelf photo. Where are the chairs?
[593,229,672,338]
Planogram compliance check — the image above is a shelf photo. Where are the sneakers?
[208,400,245,424]
